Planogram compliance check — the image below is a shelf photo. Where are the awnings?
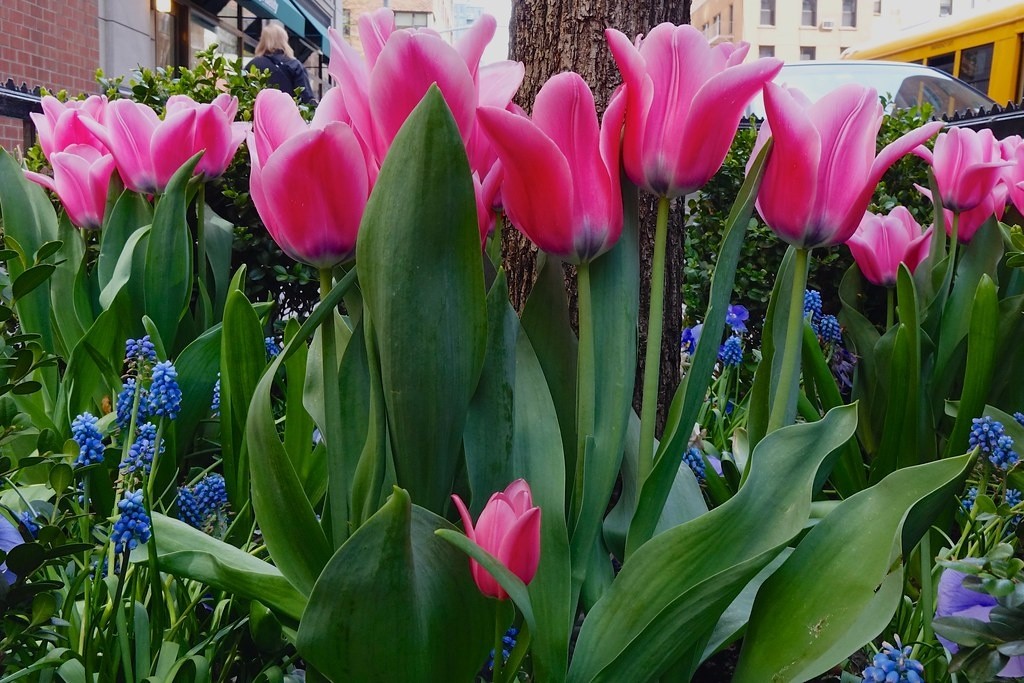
[290,0,331,59]
[236,0,306,38]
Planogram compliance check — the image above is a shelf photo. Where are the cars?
[739,59,1003,127]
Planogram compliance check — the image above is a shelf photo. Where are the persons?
[244,24,315,110]
